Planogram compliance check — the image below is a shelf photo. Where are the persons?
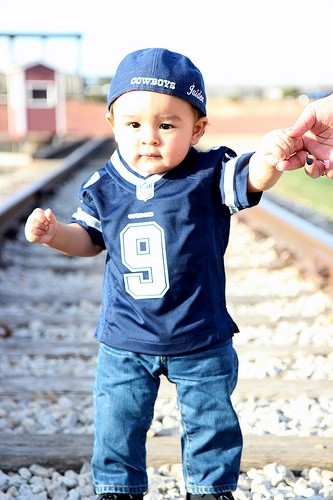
[23,47,306,500]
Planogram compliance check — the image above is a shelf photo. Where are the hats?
[106,47,209,114]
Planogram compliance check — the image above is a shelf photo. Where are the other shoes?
[185,488,235,499]
[94,489,145,500]
